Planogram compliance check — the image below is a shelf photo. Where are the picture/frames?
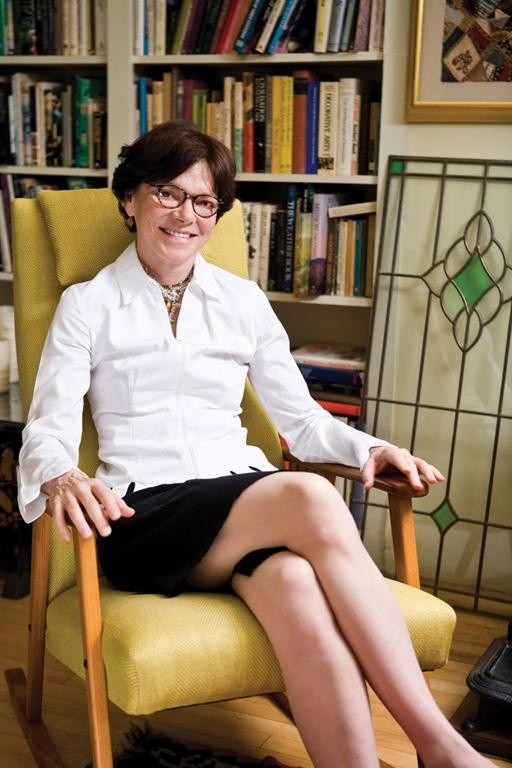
[403,0,512,123]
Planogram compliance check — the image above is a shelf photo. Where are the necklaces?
[141,263,195,323]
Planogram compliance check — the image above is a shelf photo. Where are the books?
[1,173,88,272]
[1,306,23,422]
[0,72,107,169]
[131,1,385,56]
[1,0,108,56]
[290,341,369,405]
[132,63,381,175]
[241,184,376,297]
[278,414,360,510]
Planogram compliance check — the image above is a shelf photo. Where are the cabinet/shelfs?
[1,0,388,510]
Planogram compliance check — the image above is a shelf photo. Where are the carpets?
[84,716,293,768]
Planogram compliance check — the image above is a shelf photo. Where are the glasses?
[145,180,224,218]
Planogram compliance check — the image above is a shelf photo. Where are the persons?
[16,122,497,768]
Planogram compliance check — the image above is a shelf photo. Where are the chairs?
[8,188,458,768]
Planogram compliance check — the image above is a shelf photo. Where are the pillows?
[36,189,250,287]
